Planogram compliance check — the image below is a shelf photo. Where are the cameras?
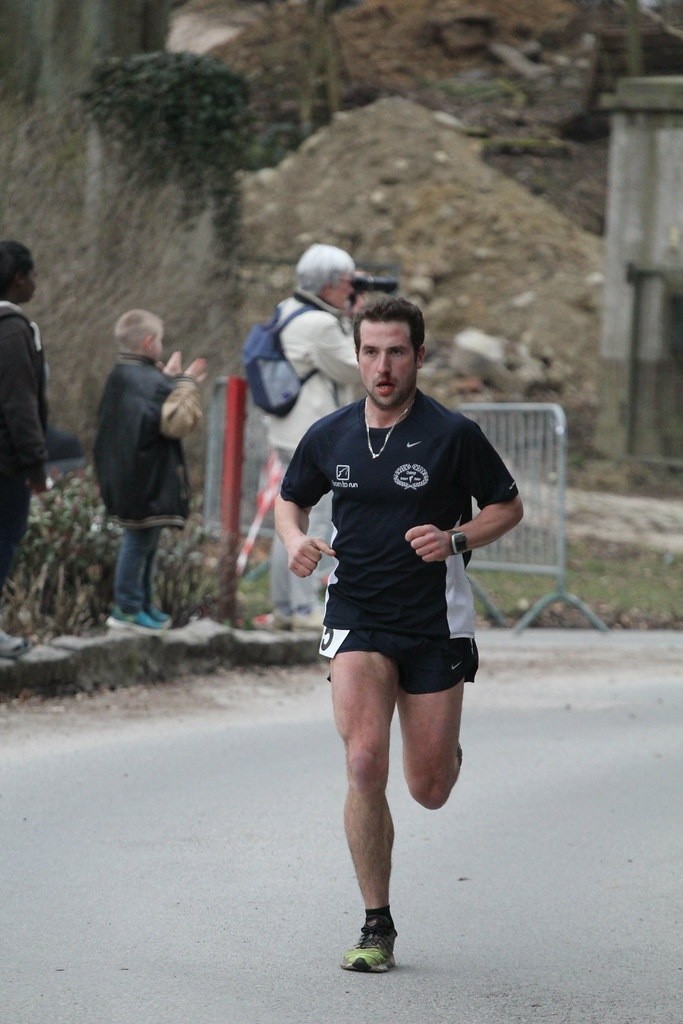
[350,275,399,306]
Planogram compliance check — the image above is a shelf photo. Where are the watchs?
[446,530,467,554]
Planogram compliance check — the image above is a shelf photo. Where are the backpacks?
[241,302,339,417]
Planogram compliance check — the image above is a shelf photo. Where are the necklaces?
[365,398,415,458]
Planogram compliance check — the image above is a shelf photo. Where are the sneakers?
[144,602,173,629]
[341,915,397,972]
[104,605,163,636]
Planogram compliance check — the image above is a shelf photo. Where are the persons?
[244,242,370,630]
[272,294,522,972]
[0,239,44,657]
[95,310,206,634]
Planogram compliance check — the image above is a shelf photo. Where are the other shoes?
[291,607,325,633]
[269,608,298,632]
[0,628,32,657]
